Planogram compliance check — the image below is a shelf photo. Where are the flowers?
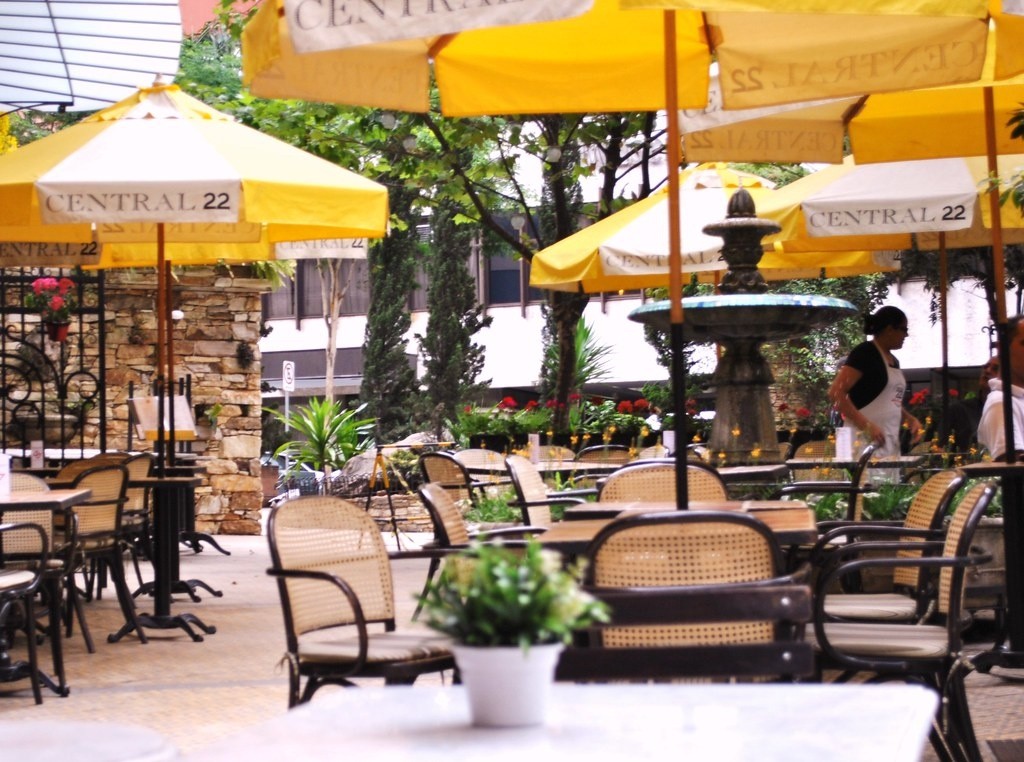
[25,277,77,322]
[411,542,614,647]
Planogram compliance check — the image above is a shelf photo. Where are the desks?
[539,463,789,484]
[534,507,817,567]
[12,466,222,604]
[562,498,809,517]
[788,452,930,478]
[0,449,231,557]
[0,487,93,673]
[41,477,217,644]
[194,676,941,762]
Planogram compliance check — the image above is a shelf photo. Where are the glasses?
[898,326,908,332]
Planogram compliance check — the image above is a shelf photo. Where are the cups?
[836,427,852,461]
[30,440,43,469]
[663,430,675,454]
[0,454,11,498]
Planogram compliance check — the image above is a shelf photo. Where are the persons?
[974,314,1024,464]
[950,354,1001,459]
[826,304,922,486]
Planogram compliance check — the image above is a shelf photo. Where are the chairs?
[0,449,155,704]
[265,443,998,762]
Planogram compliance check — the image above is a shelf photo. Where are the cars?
[261,451,323,497]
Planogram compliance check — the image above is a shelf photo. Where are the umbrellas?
[525,69,1022,464]
[1,73,389,642]
[243,1,1023,514]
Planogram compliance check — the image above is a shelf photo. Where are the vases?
[45,319,72,341]
[444,642,565,728]
